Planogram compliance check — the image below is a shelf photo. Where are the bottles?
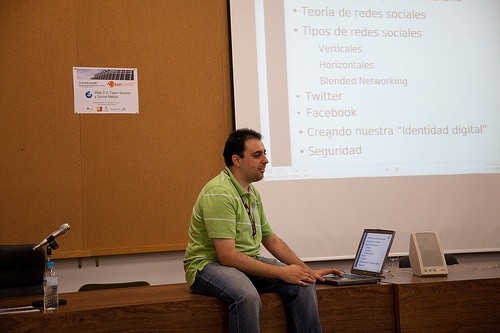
[44,262,59,310]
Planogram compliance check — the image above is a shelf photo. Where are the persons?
[183,128,344,333]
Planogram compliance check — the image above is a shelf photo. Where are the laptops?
[316,229,396,286]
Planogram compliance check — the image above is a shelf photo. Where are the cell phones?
[32,299,67,307]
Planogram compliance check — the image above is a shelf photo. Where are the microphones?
[32,223,71,252]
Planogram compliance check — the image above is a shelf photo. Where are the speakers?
[408,231,449,277]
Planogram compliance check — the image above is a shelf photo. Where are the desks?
[0,263,500,333]
